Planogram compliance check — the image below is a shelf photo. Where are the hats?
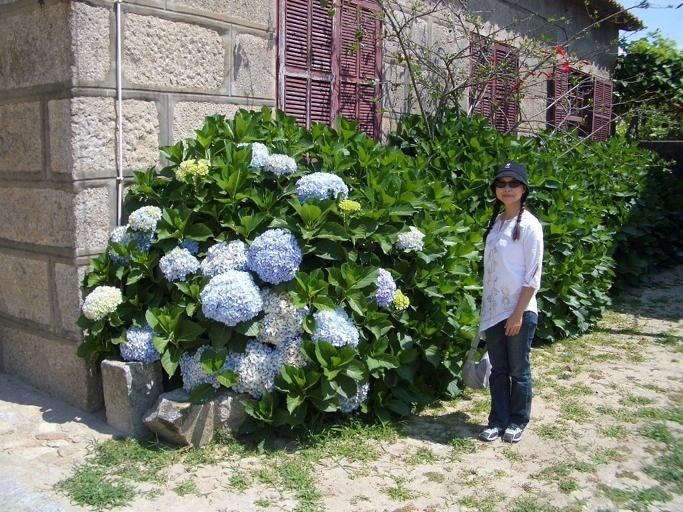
[490,162,530,198]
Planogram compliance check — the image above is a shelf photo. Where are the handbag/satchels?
[462,352,492,388]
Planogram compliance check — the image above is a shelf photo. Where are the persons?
[477,162,544,443]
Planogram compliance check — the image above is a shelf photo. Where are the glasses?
[495,180,521,188]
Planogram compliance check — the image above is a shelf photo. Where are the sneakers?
[479,426,506,441]
[503,422,524,442]
